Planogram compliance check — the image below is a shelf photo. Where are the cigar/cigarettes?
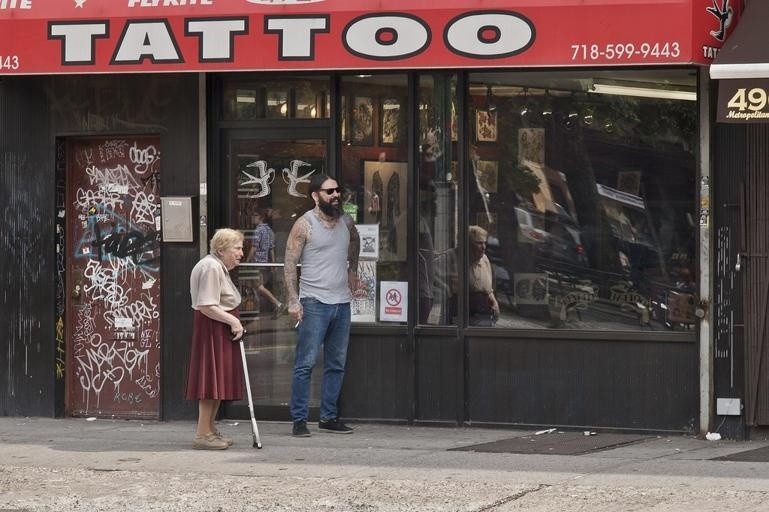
[295,320,300,328]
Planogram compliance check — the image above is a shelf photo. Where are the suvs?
[514,205,582,270]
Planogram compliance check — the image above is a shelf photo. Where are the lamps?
[485,84,497,111]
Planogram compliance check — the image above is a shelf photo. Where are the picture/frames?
[616,171,642,198]
[360,158,410,265]
[321,90,403,149]
[474,107,547,245]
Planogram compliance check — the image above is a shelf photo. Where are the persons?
[188,229,245,449]
[385,174,439,324]
[246,208,287,320]
[266,206,288,311]
[283,172,362,437]
[432,224,499,326]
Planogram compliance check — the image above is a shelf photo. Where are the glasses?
[320,187,342,195]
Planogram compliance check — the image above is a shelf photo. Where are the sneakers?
[271,303,288,319]
[192,430,233,450]
[293,420,311,437]
[318,418,354,434]
